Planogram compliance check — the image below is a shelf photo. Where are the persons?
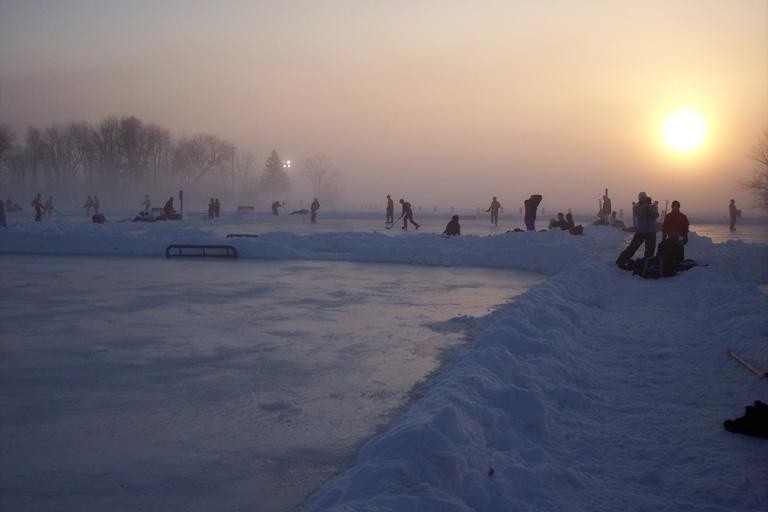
[215,198,220,216]
[385,195,394,223]
[486,197,500,226]
[32,193,55,223]
[164,197,173,218]
[272,201,281,215]
[142,194,151,215]
[525,194,542,232]
[400,199,420,230]
[729,200,740,230]
[549,191,688,265]
[446,215,460,235]
[208,199,214,219]
[84,195,99,216]
[0,197,22,227]
[311,198,320,222]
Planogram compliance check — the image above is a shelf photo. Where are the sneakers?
[723,400,767,440]
